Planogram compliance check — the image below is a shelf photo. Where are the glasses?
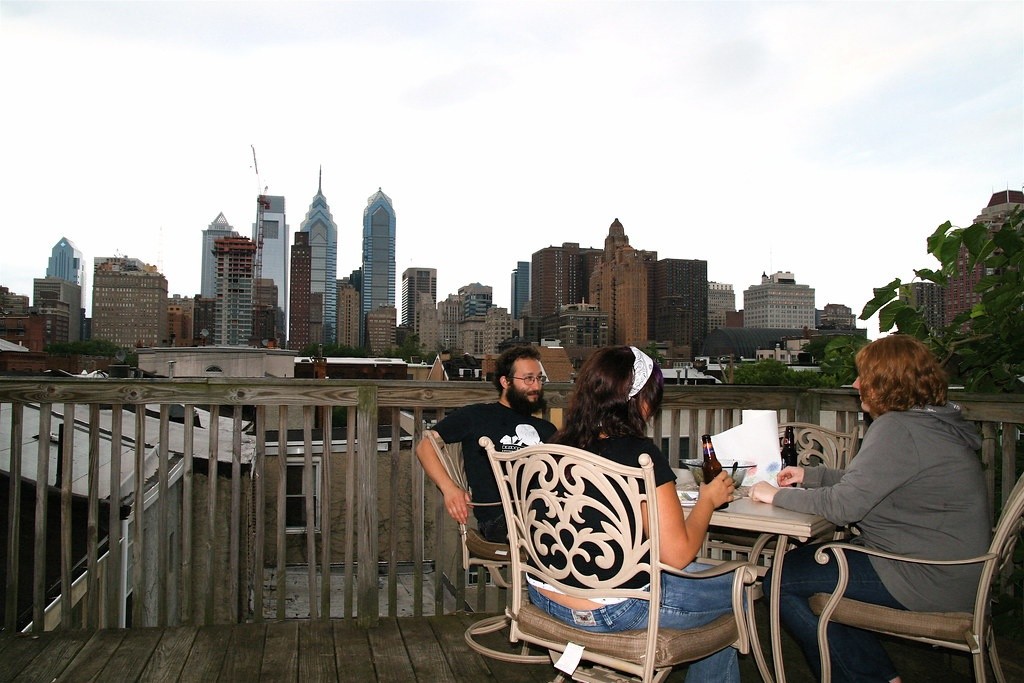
[509,374,547,385]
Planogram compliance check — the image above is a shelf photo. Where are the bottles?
[781,425,798,487]
[702,435,729,511]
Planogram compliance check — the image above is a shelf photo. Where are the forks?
[681,493,698,501]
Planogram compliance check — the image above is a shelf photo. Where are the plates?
[676,490,701,507]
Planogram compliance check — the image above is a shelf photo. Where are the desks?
[671,482,842,683]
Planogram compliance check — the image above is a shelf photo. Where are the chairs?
[422,422,1024,683]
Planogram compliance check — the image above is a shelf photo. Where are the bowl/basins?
[681,459,757,489]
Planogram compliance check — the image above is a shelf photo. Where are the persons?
[524,345,748,683]
[749,334,991,683]
[417,346,557,544]
